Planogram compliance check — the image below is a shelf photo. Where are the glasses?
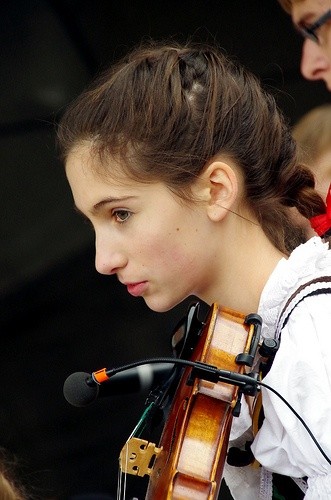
[299,7,331,48]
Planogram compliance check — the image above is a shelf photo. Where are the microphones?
[63,372,98,407]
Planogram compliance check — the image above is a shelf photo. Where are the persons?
[57,41,331,500]
[280,0,331,90]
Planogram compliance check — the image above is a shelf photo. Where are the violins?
[146,304,261,499]
